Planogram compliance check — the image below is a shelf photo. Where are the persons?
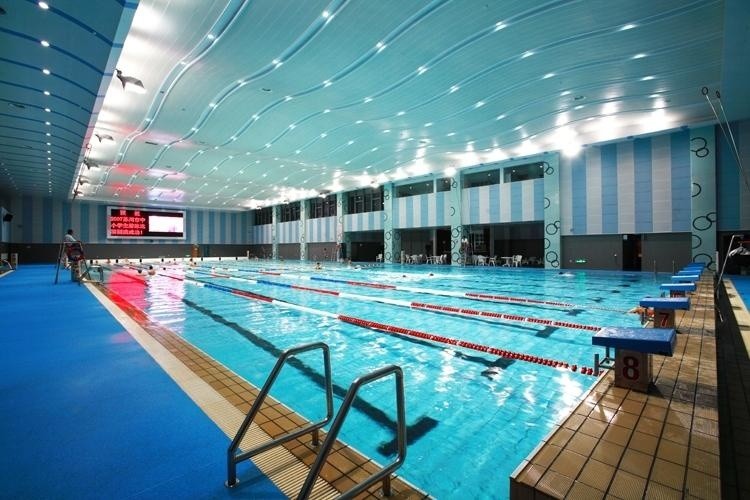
[314,262,322,270]
[323,247,328,261]
[96,257,216,276]
[628,296,654,318]
[64,228,86,259]
[461,235,468,250]
[347,257,352,264]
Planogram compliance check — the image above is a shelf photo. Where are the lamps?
[376,254,523,268]
